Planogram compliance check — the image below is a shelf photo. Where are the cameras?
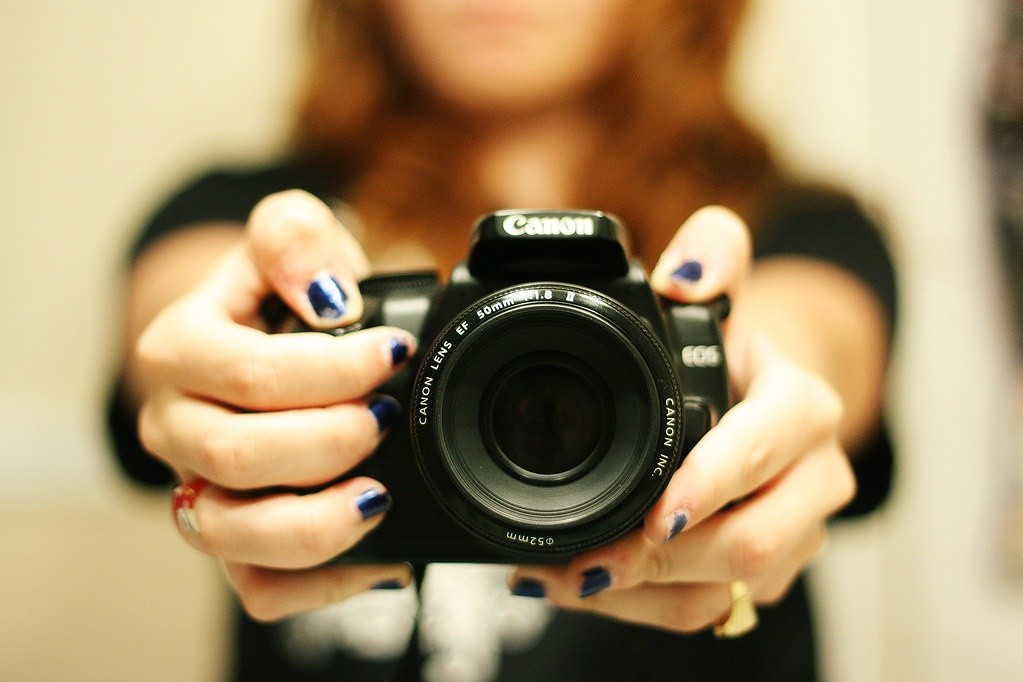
[280,206,733,570]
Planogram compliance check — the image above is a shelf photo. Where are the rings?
[714,581,759,637]
[174,478,211,557]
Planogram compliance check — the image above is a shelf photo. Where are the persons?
[107,1,896,682]
